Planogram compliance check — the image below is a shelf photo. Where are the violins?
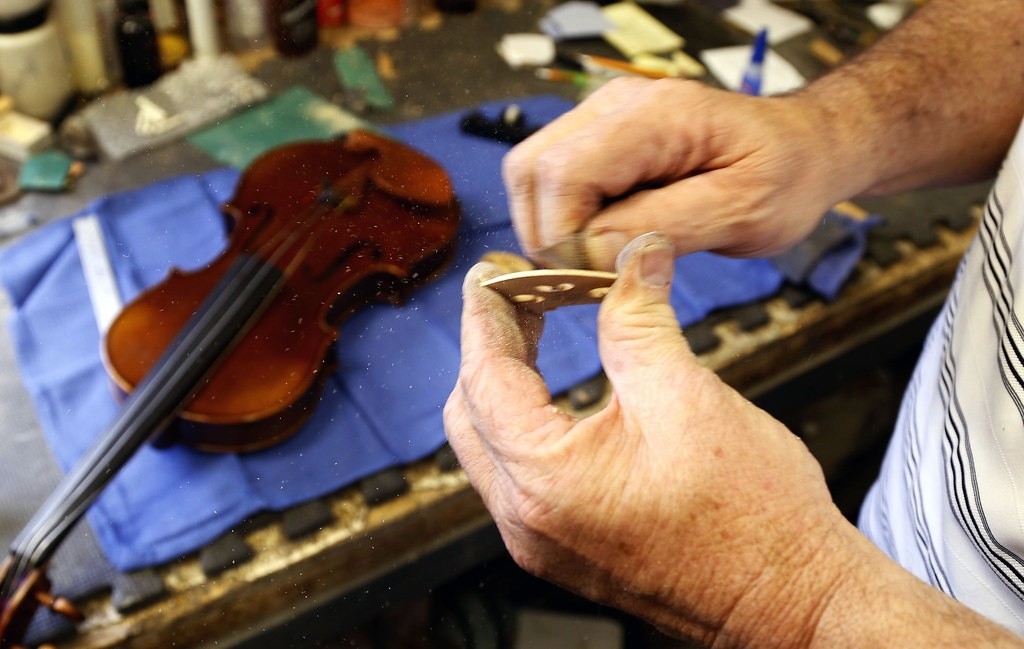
[0,128,461,649]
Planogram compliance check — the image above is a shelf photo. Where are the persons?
[440,0,1024,648]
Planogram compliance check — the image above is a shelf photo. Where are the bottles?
[0,0,402,121]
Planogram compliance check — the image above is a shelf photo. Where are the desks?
[0,0,992,649]
